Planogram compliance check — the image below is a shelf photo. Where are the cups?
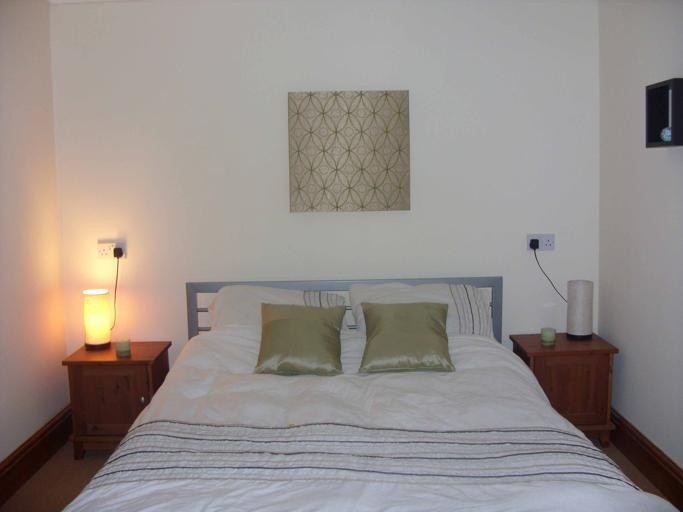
[115,339,131,358]
[540,328,557,347]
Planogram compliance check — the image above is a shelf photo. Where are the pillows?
[256,303,346,377]
[208,286,348,330]
[351,282,491,335]
[359,302,456,375]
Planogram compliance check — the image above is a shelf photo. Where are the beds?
[63,276,681,511]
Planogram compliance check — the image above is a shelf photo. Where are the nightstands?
[510,333,618,451]
[64,341,173,459]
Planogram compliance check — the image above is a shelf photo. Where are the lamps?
[82,289,113,349]
[566,280,595,340]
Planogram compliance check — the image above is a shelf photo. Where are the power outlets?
[526,232,554,250]
[99,242,126,258]
